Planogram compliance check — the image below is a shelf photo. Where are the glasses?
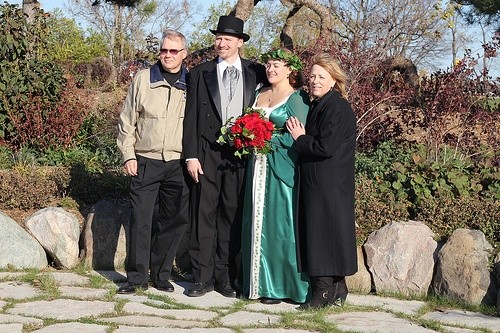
[159,46,185,55]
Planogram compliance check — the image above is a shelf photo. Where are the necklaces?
[268,85,291,102]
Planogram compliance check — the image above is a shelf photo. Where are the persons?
[115,31,189,294]
[285,53,359,313]
[179,16,268,297]
[247,49,307,304]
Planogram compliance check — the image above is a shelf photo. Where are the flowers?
[216,106,278,158]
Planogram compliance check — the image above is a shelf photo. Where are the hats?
[209,16,250,42]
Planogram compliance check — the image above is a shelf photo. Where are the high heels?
[295,276,348,311]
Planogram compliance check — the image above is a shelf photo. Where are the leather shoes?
[117,281,148,293]
[188,279,215,297]
[150,277,174,292]
[216,280,242,298]
